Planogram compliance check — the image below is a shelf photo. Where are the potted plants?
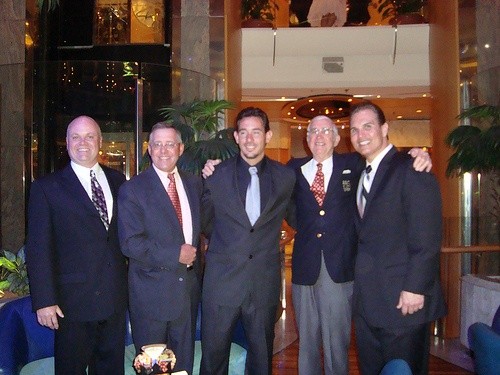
[443,105,500,345]
[378,0,423,23]
[241,0,279,27]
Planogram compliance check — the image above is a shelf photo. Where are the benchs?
[0,293,251,375]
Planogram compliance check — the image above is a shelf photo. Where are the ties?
[244,167,261,227]
[310,163,325,207]
[166,173,183,229]
[357,165,373,218]
[90,170,109,227]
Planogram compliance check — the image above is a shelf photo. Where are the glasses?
[150,142,179,150]
[309,127,335,136]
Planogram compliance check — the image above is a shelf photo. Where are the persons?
[351,102,449,375]
[198,108,297,375]
[117,122,204,375]
[202,115,432,375]
[25,116,127,375]
